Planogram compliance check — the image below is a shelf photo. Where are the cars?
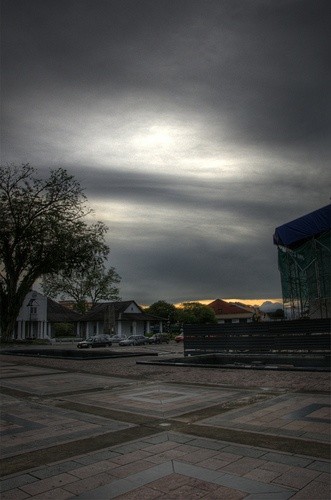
[174,331,184,343]
[119,335,149,346]
[108,334,126,343]
[77,336,112,349]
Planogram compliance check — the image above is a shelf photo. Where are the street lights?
[26,298,36,339]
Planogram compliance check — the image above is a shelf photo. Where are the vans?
[150,333,170,344]
